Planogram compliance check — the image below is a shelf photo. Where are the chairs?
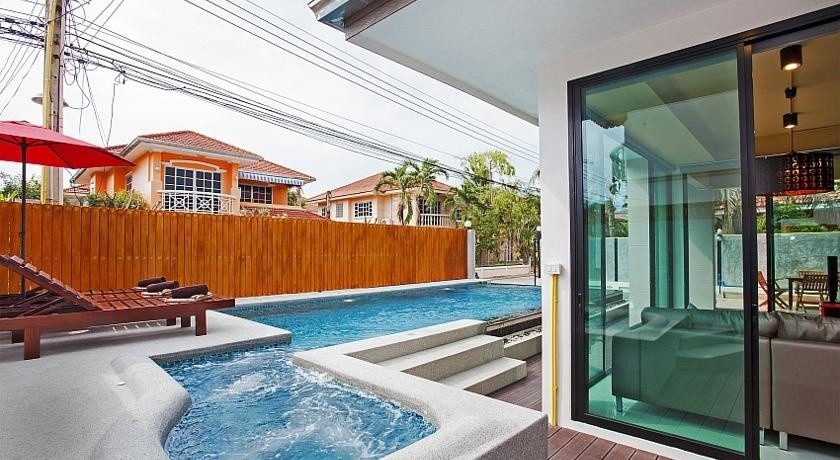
[758,270,840,313]
[0,253,237,365]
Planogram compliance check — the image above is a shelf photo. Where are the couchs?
[611,306,840,451]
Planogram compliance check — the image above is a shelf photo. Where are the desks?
[786,275,828,310]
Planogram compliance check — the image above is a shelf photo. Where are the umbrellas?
[0,120,137,300]
